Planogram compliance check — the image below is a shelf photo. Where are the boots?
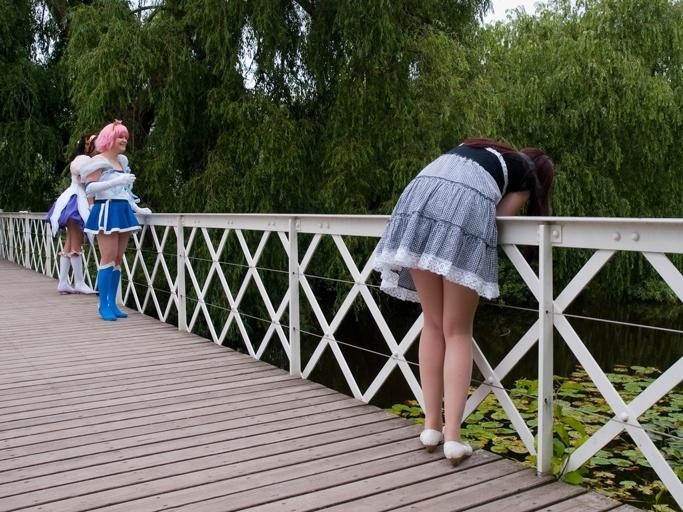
[97,263,117,322]
[69,251,98,295]
[108,265,128,318]
[57,251,78,295]
[85,172,136,199]
[128,201,153,216]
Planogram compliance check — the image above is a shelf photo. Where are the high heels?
[419,429,444,453]
[443,441,473,467]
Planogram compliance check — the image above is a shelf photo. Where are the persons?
[372,136,556,464]
[44,135,99,294]
[78,119,151,320]
